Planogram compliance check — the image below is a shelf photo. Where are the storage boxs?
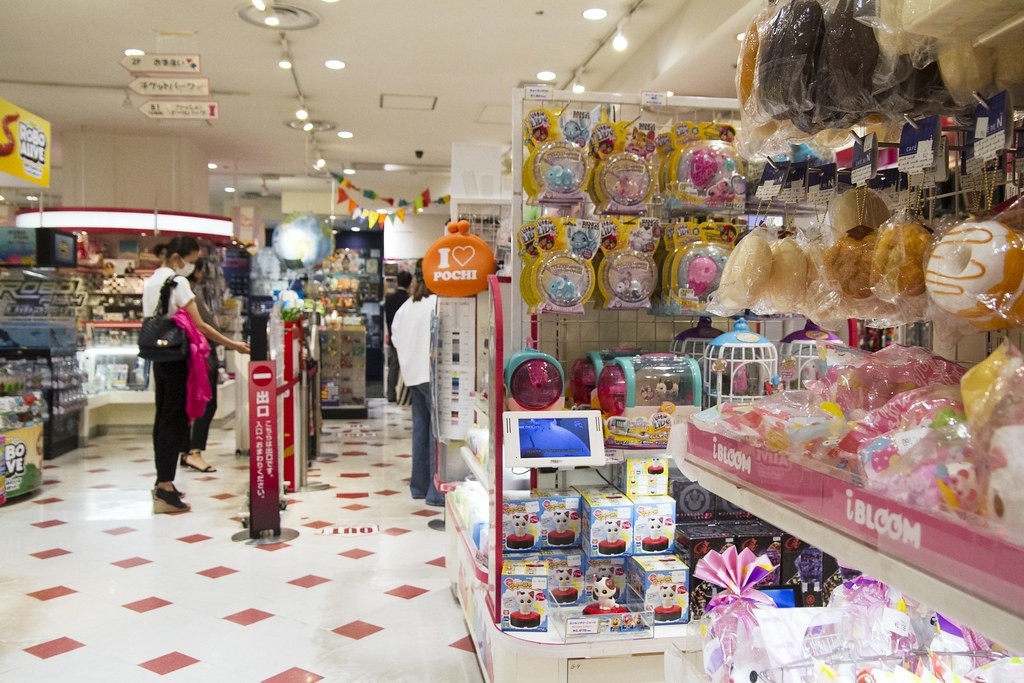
[501,459,824,628]
[436,443,477,484]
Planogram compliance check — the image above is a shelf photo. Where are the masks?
[172,257,196,278]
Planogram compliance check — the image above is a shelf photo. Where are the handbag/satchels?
[137,274,189,362]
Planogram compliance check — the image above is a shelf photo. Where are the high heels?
[152,479,186,500]
[153,488,191,514]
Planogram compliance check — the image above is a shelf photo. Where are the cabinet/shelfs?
[663,400,1024,683]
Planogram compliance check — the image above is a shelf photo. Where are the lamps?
[297,93,309,121]
[279,50,295,71]
[573,65,586,92]
[613,15,632,50]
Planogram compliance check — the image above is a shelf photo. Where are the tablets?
[503,410,606,467]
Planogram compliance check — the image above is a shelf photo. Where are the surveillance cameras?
[415,150,423,159]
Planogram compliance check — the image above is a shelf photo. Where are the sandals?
[180,452,217,472]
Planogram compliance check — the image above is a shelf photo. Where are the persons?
[185,258,218,472]
[384,271,412,402]
[142,235,252,513]
[390,259,446,506]
